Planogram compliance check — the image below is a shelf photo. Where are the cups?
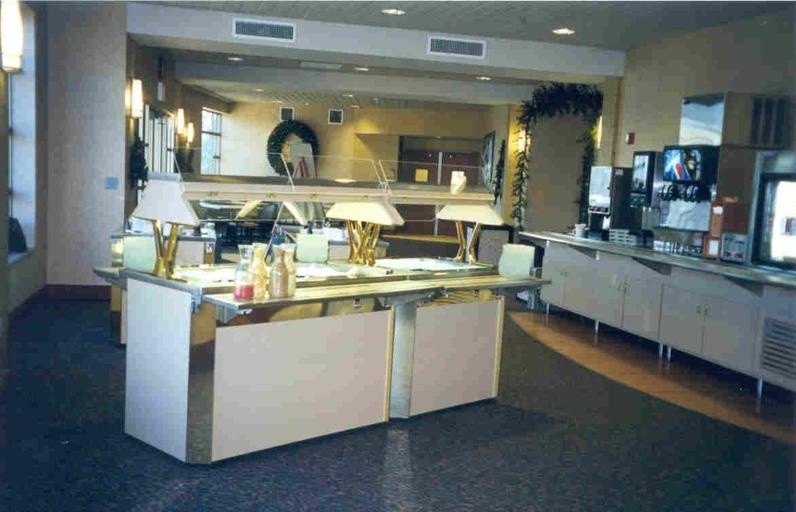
[573,224,585,237]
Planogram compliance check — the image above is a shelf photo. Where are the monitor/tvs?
[751,172,796,270]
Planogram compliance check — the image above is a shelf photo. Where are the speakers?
[279,107,295,123]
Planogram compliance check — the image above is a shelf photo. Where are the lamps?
[131,78,144,119]
[175,107,185,135]
[186,122,195,142]
[1,0,23,73]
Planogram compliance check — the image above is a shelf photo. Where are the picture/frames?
[280,106,295,122]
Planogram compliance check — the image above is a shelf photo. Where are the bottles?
[235,242,295,299]
